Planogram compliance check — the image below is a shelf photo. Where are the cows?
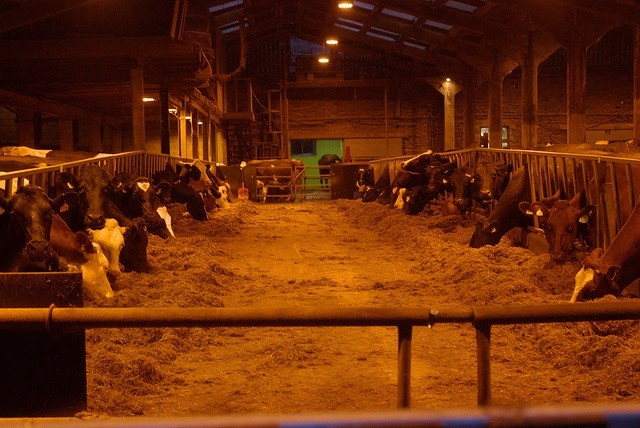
[216,166,232,203]
[0,160,131,230]
[318,154,343,191]
[146,201,177,240]
[0,185,77,272]
[530,190,597,264]
[414,158,457,194]
[204,170,231,211]
[0,172,132,281]
[401,149,449,176]
[517,188,560,226]
[239,158,304,204]
[351,166,374,199]
[103,197,150,274]
[165,161,209,222]
[0,146,172,223]
[403,185,446,215]
[470,149,640,249]
[361,162,391,203]
[392,185,416,210]
[568,199,640,303]
[462,139,640,206]
[152,170,180,205]
[436,160,481,211]
[0,205,116,307]
[176,159,213,188]
